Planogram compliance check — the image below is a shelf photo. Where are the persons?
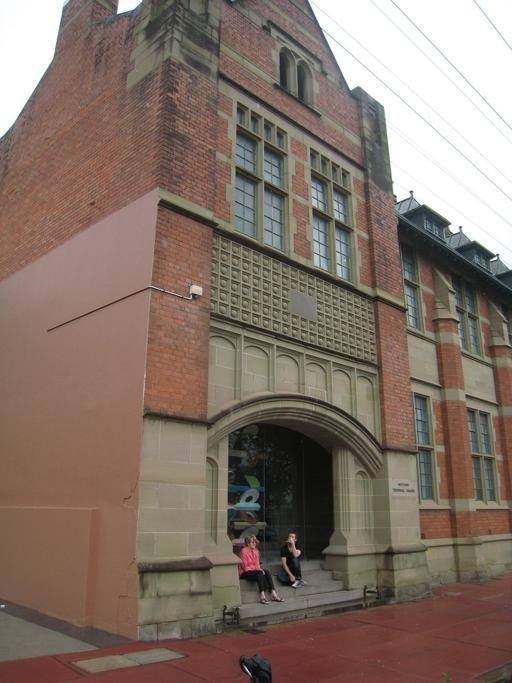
[280,531,304,588]
[239,534,284,604]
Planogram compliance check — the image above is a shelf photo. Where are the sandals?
[260,597,270,603]
[271,596,285,601]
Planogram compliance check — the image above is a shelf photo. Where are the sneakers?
[292,579,301,586]
